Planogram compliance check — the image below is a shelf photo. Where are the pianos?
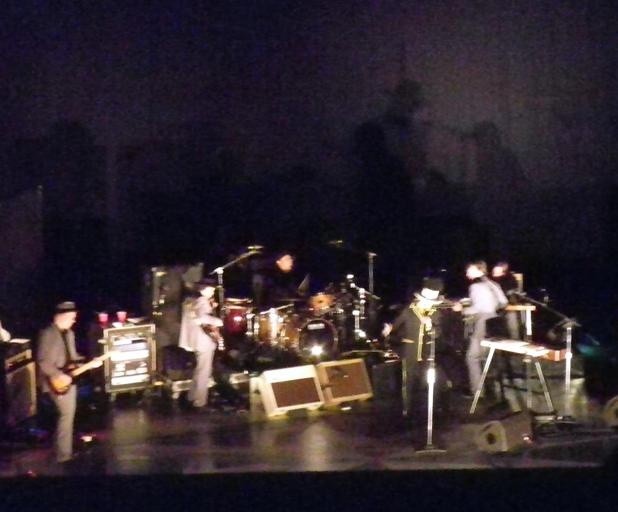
[479,336,573,362]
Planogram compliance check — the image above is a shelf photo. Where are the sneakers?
[187,399,223,413]
[459,390,486,399]
[58,450,79,466]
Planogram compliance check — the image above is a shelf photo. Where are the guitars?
[47,348,122,395]
[423,296,474,319]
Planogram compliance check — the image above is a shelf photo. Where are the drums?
[254,308,294,346]
[277,313,340,363]
[306,291,337,317]
[221,297,250,310]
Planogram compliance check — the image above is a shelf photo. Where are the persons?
[30,298,107,468]
[448,258,510,401]
[481,261,521,295]
[175,277,226,413]
[381,272,446,410]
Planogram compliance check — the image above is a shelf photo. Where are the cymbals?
[260,302,296,314]
[274,296,308,302]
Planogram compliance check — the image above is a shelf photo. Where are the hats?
[193,278,217,284]
[413,287,443,304]
[56,301,79,312]
